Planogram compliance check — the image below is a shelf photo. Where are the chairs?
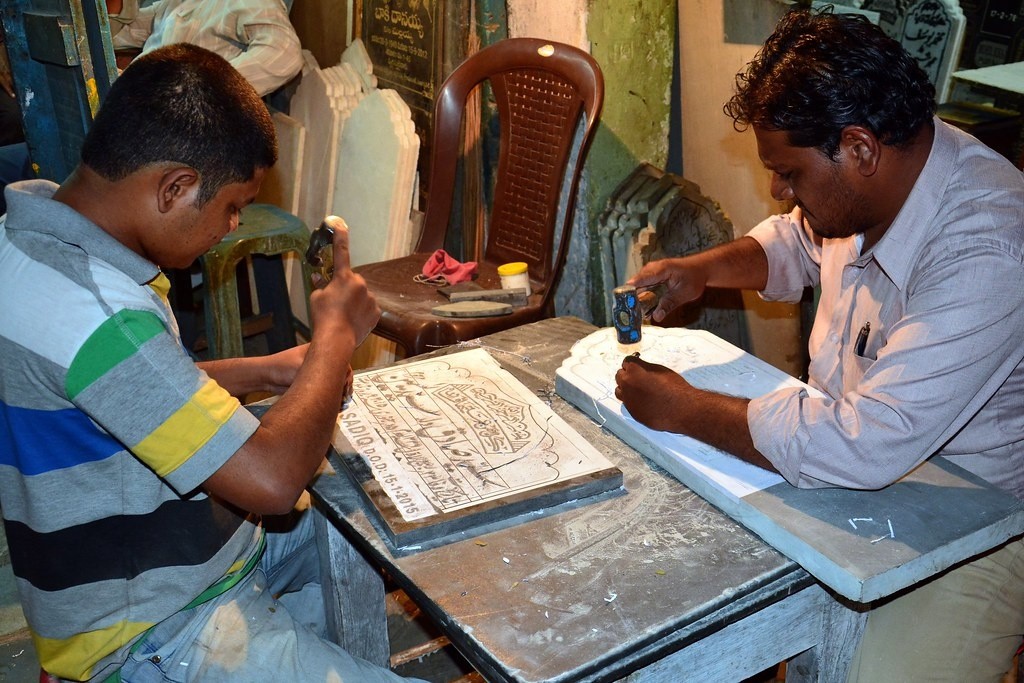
[311,37,604,357]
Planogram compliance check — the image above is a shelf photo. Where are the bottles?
[497,262,532,296]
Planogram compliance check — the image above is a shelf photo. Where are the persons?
[0,44,433,682]
[0,0,301,203]
[613,9,1024,682]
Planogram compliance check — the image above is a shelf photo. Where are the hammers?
[612,281,668,345]
[304,215,348,283]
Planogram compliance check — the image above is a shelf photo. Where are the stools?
[195,201,315,359]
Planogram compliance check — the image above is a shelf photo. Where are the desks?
[236,315,871,683]
